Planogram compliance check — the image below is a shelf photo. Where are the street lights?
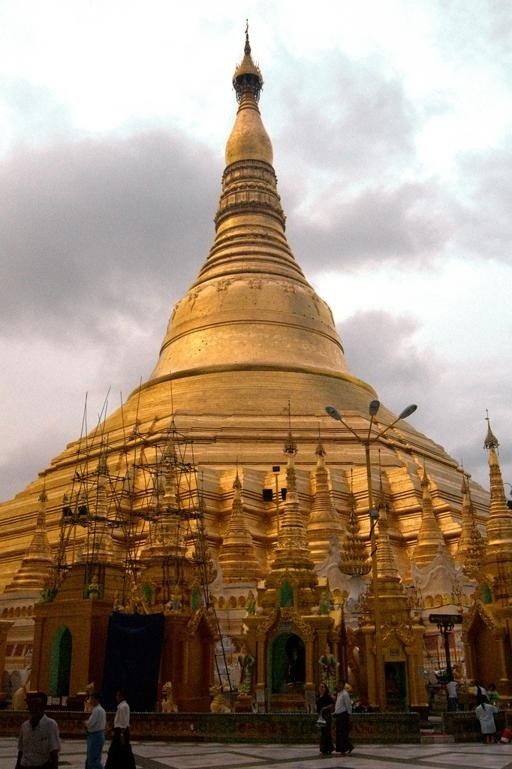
[321,398,420,713]
[428,613,463,686]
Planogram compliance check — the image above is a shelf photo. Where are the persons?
[335,683,355,755]
[316,683,335,755]
[15,693,62,769]
[238,643,255,694]
[89,575,100,599]
[470,680,501,744]
[104,689,136,769]
[446,676,458,712]
[319,644,340,696]
[81,692,106,769]
